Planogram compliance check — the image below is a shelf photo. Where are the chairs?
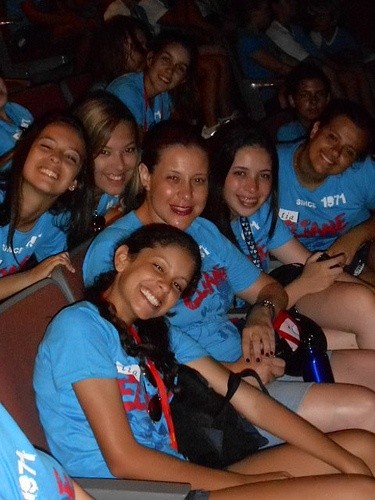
[0,28,297,500]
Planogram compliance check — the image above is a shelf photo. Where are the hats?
[225,305,330,378]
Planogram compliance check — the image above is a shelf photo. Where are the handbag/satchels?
[164,362,269,470]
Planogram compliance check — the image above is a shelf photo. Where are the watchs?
[246,300,277,322]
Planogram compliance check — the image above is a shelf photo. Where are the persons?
[259,61,331,144]
[83,119,375,452]
[0,0,375,127]
[33,223,374,500]
[0,111,95,305]
[67,90,143,237]
[103,24,202,141]
[270,98,374,292]
[200,115,375,350]
[0,77,36,173]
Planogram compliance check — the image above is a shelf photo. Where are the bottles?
[300,336,334,383]
